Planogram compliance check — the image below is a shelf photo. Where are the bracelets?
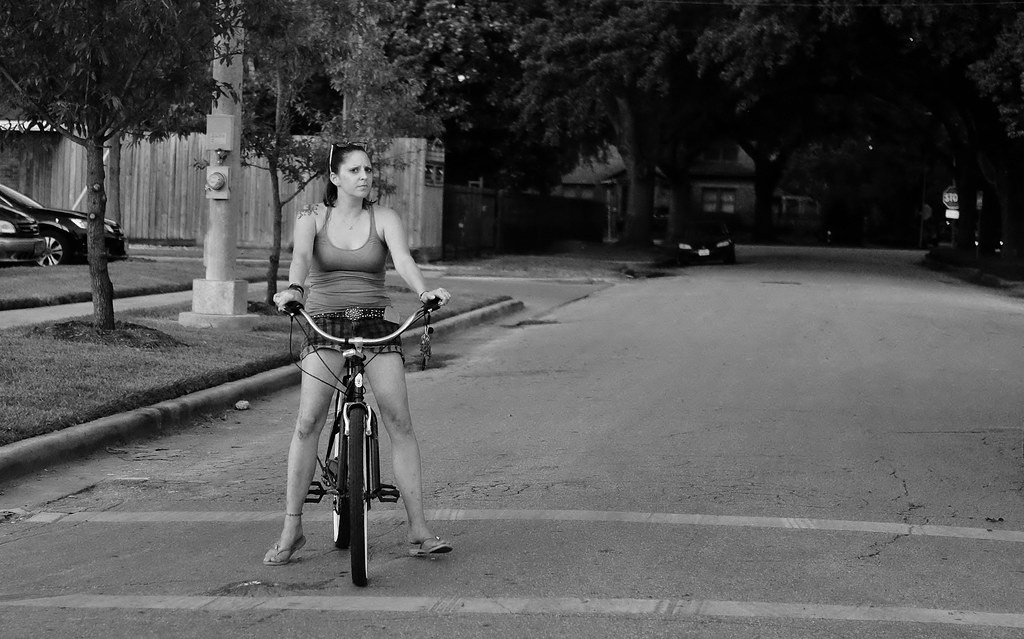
[287,283,305,299]
[262,140,454,568]
[418,290,430,305]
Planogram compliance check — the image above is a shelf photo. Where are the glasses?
[329,141,368,173]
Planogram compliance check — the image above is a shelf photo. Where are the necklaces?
[334,206,363,231]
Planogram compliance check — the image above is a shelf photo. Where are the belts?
[310,307,385,321]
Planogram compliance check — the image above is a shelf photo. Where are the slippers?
[408,536,453,556]
[263,534,306,565]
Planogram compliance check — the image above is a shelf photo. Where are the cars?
[0,182,131,269]
[676,219,736,266]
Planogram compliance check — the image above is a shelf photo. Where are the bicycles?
[284,296,441,586]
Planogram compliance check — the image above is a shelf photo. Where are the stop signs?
[942,186,960,209]
